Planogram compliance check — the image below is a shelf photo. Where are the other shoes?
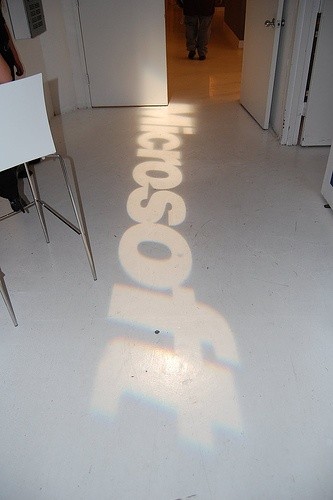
[198,56,206,61]
[188,51,195,60]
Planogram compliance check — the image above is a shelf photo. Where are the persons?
[178,0,214,61]
[0,56,33,214]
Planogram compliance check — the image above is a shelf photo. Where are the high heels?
[9,196,26,213]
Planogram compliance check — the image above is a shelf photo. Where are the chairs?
[0,72,97,326]
